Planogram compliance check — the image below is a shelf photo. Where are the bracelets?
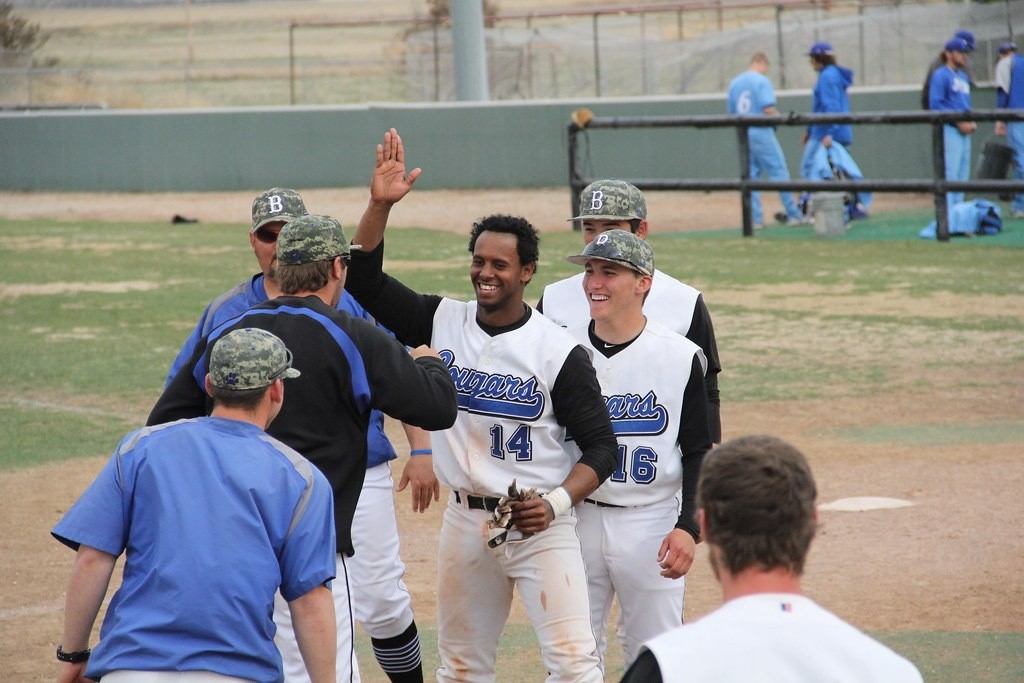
[56,645,90,662]
[542,486,572,521]
[410,448,432,456]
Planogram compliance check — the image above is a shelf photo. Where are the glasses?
[269,348,293,380]
[257,229,278,244]
[324,254,351,266]
[580,241,652,277]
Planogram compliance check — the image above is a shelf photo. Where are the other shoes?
[1007,209,1024,218]
[753,221,767,230]
[775,213,787,224]
[787,216,815,226]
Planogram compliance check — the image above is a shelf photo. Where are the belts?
[455,490,501,513]
[585,498,636,508]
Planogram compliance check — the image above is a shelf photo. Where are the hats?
[566,177,647,220]
[802,42,832,56]
[251,187,309,235]
[955,29,976,49]
[210,328,302,389]
[566,229,655,278]
[276,212,363,266]
[998,40,1018,55]
[945,38,971,51]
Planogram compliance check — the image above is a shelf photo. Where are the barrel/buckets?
[812,191,845,233]
[975,133,1016,179]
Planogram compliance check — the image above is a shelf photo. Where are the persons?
[167,187,442,683]
[558,229,710,683]
[993,42,1024,218]
[51,328,337,683]
[728,51,817,230]
[619,433,926,683]
[536,178,721,448]
[920,31,978,208]
[146,213,458,683]
[345,126,623,683]
[774,42,869,223]
